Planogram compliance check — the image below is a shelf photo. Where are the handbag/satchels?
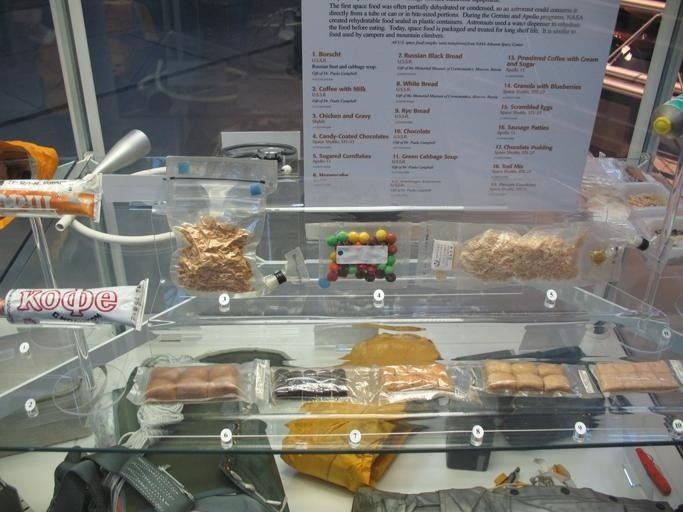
[48,420,292,512]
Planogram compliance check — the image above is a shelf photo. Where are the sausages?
[636,448,671,495]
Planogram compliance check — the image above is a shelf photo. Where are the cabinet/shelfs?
[0,205,683,510]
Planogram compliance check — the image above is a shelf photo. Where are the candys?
[318,229,398,288]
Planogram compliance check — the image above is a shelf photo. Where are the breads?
[382,362,453,392]
[596,360,679,393]
[274,368,348,400]
[146,364,239,400]
[484,359,571,393]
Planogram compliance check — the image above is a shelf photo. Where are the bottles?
[649,93,683,137]
[609,210,650,251]
[81,129,153,175]
